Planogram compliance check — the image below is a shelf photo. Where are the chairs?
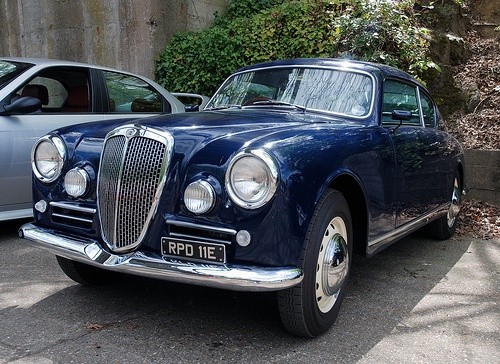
[61,85,88,112]
[22,84,48,112]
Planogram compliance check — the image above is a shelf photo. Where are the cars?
[18,55,465,341]
[1,56,211,228]
